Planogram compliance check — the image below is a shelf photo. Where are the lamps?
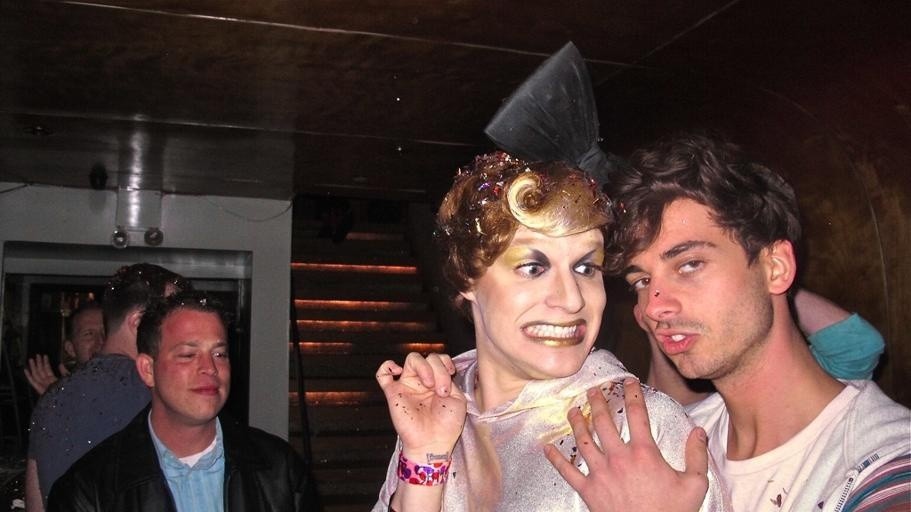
[110,226,164,251]
